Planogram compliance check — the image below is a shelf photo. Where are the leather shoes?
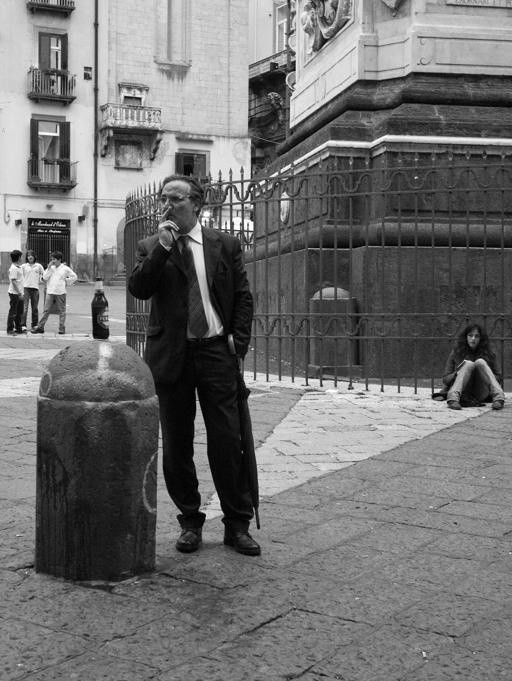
[223,526,260,556]
[175,526,203,552]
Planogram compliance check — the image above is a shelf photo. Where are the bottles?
[90,276,110,339]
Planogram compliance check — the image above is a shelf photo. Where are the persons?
[30,250,78,335]
[5,250,27,337]
[126,173,262,559]
[21,248,44,331]
[430,323,505,412]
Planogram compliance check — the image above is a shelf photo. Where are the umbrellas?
[227,334,261,532]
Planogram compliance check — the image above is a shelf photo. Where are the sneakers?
[493,400,505,409]
[7,325,65,335]
[449,400,461,409]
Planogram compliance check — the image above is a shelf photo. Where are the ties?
[179,236,209,338]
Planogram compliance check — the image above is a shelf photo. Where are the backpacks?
[431,371,485,406]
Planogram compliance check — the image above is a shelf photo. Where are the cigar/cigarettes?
[156,215,165,218]
